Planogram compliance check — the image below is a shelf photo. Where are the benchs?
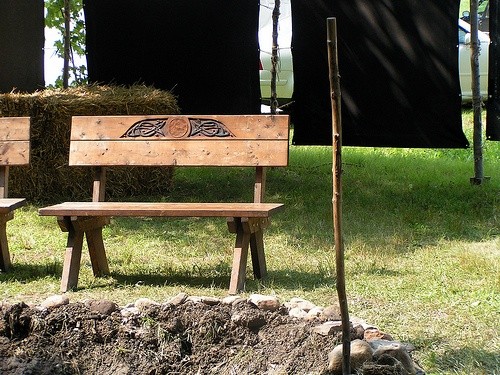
[39,115,290,295]
[0,116,31,270]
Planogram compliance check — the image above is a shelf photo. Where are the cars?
[460,0,490,31]
[459,19,489,105]
